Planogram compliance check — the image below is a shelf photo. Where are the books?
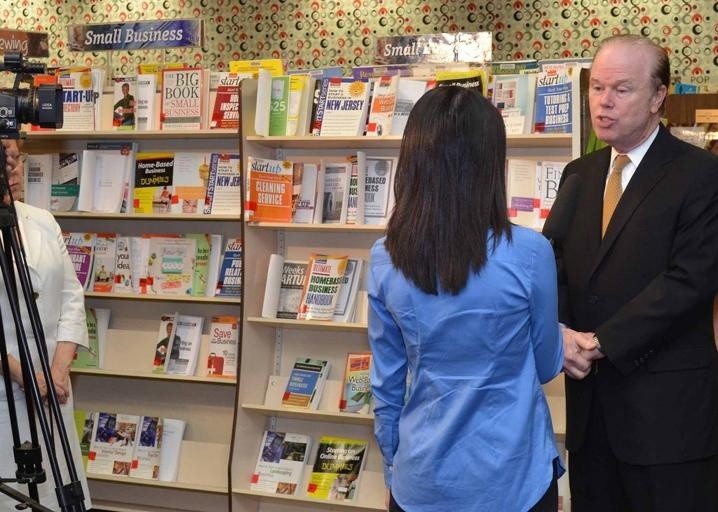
[114,72,157,132]
[72,408,187,485]
[161,66,209,131]
[671,127,706,148]
[278,355,332,411]
[211,70,251,133]
[67,307,110,370]
[21,140,242,221]
[69,304,240,382]
[153,312,204,378]
[249,428,311,497]
[244,154,294,223]
[346,148,399,227]
[304,435,369,503]
[206,313,238,380]
[290,161,326,222]
[321,162,352,224]
[57,229,243,301]
[259,250,367,322]
[339,349,373,420]
[57,67,106,131]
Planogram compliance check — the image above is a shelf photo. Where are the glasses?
[3,152,28,163]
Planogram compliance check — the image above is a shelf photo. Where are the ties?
[602,155,631,241]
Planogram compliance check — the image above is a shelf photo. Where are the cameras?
[0,52,64,140]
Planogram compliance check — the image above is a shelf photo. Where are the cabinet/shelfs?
[585,92,718,164]
[247,62,583,511]
[11,68,241,512]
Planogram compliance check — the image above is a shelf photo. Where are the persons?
[366,86,566,511]
[156,323,180,361]
[542,33,717,512]
